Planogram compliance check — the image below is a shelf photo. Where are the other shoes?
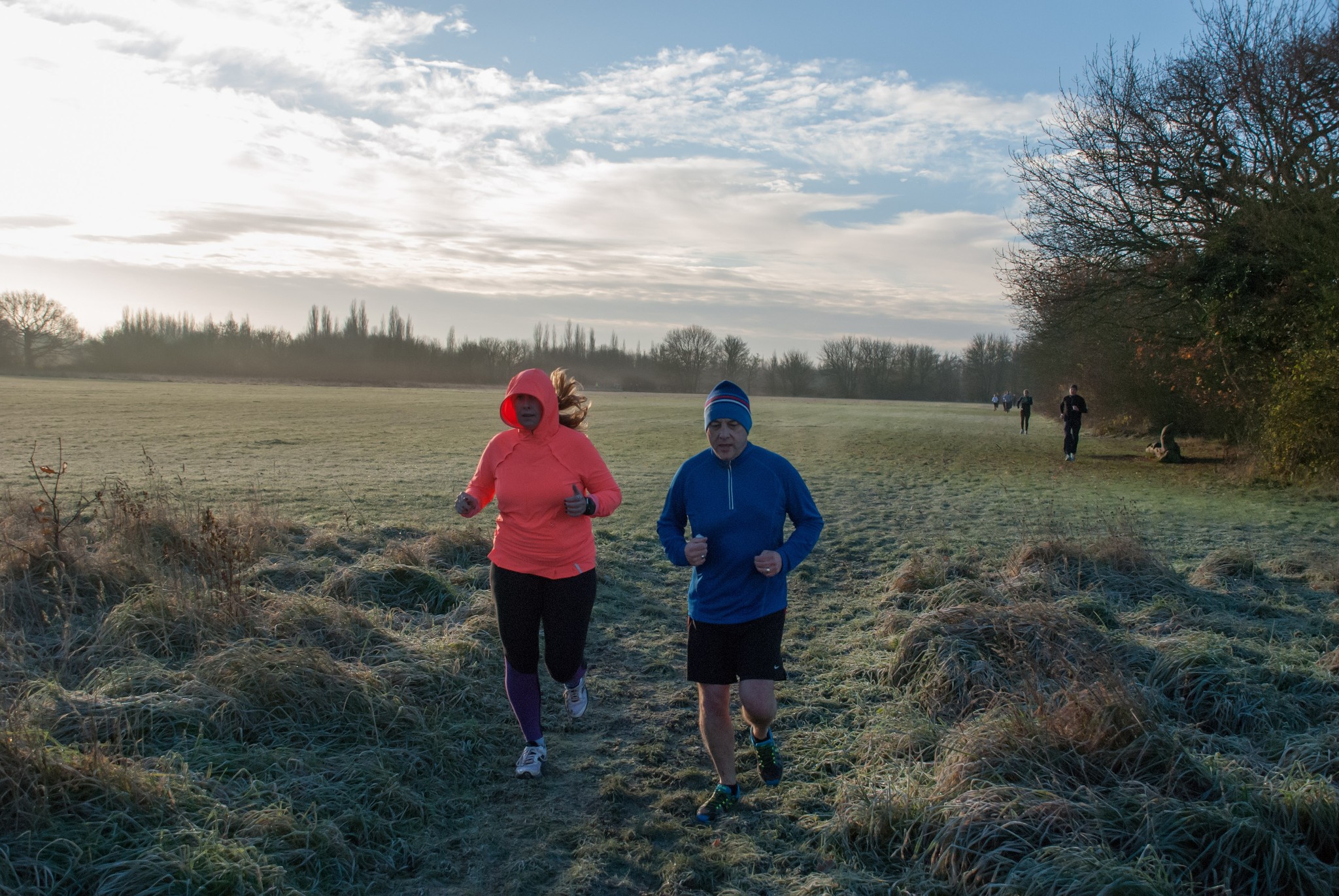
[1025,431,1028,435]
[1020,429,1024,434]
[1070,453,1075,461]
[1065,455,1070,461]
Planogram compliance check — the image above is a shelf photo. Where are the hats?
[704,380,753,436]
[1069,384,1078,391]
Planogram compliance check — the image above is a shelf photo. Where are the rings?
[766,567,770,574]
[702,555,704,559]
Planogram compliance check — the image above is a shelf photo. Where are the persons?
[992,390,1016,413]
[1017,389,1033,434]
[657,380,824,823]
[454,368,622,778]
[1059,384,1087,461]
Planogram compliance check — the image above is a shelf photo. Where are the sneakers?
[750,724,783,785]
[515,739,547,779]
[563,672,588,718]
[696,781,740,821]
